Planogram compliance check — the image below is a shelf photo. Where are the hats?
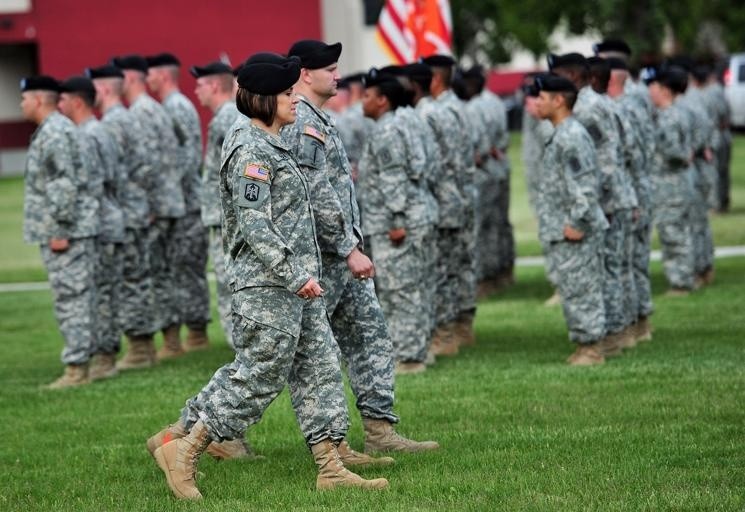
[343,73,365,81]
[608,57,628,69]
[188,61,236,78]
[86,65,125,78]
[369,66,416,101]
[147,53,181,67]
[337,82,349,88]
[61,76,96,92]
[421,54,457,67]
[20,75,62,91]
[546,52,585,67]
[363,73,404,109]
[236,51,302,96]
[586,56,615,71]
[113,55,148,71]
[597,39,632,56]
[288,39,343,70]
[522,84,542,98]
[404,63,435,76]
[534,73,579,92]
[642,60,685,93]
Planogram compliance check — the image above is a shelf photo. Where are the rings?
[360,275,365,278]
[304,295,308,299]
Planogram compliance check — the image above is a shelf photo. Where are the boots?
[86,350,119,380]
[462,331,475,346]
[504,274,511,288]
[454,332,464,347]
[476,284,487,300]
[241,435,268,461]
[566,343,583,362]
[179,323,187,356]
[206,438,257,464]
[337,437,396,467]
[485,280,496,294]
[148,336,162,366]
[115,338,151,370]
[43,363,89,391]
[146,416,190,455]
[511,273,515,284]
[182,328,207,351]
[496,279,506,292]
[205,328,212,350]
[421,349,437,367]
[151,416,213,503]
[154,324,183,361]
[640,316,653,342]
[571,344,606,366]
[311,438,392,492]
[391,361,427,373]
[361,414,441,455]
[429,336,459,356]
[623,325,639,349]
[603,335,624,356]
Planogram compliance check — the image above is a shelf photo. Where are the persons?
[370,65,441,368]
[18,72,117,391]
[664,66,720,286]
[531,72,608,369]
[205,38,440,462]
[145,52,210,351]
[467,63,517,288]
[339,74,375,136]
[106,53,194,360]
[449,71,493,301]
[232,64,242,100]
[591,37,657,343]
[188,61,252,352]
[463,70,507,296]
[323,78,371,184]
[643,76,708,293]
[682,67,718,215]
[706,62,732,214]
[56,75,126,382]
[418,54,480,351]
[355,75,431,375]
[402,62,467,357]
[584,55,638,350]
[545,52,633,358]
[83,65,162,372]
[520,82,563,307]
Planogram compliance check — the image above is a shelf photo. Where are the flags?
[373,0,458,66]
